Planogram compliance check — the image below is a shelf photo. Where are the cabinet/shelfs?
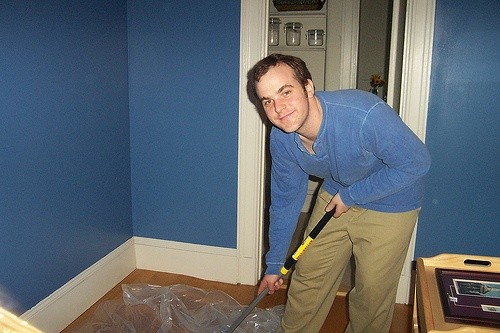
[269,0,327,95]
[412,253,500,332]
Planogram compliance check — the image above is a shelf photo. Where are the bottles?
[284,20,303,46]
[269,17,282,46]
[305,28,326,46]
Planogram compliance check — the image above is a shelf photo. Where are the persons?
[245,52,433,332]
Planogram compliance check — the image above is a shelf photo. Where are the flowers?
[370,71,385,90]
